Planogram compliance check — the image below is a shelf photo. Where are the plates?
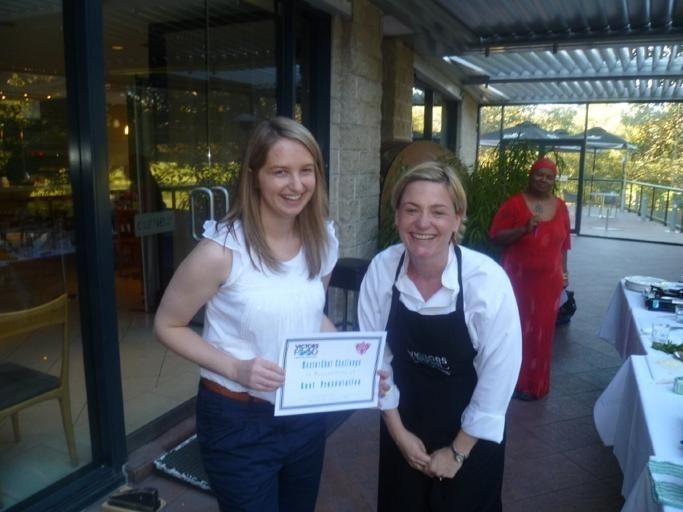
[659,281,683,291]
[624,274,669,293]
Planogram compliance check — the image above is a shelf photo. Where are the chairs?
[1,289,79,474]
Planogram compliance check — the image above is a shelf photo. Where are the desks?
[328,256,371,332]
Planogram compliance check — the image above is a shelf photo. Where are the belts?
[200,378,267,404]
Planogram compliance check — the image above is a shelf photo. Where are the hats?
[530,159,558,176]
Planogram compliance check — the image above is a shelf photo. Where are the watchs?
[450,441,471,463]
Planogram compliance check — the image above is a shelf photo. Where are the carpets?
[149,435,212,499]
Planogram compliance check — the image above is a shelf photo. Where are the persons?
[153,115,393,512]
[124,153,168,250]
[356,161,524,511]
[489,155,573,402]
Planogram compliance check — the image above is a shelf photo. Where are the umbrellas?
[480,121,560,147]
[556,125,640,218]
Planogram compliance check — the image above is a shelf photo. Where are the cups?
[651,317,671,344]
[675,304,683,323]
